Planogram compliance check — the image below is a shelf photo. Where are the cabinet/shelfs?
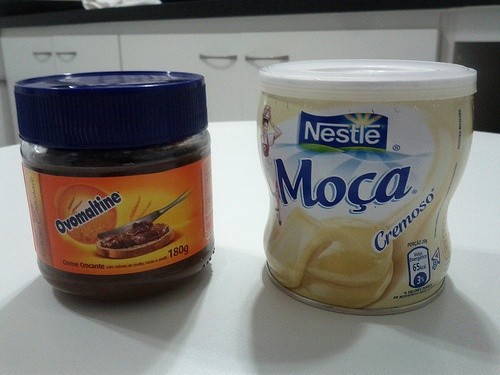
[0,0,500,143]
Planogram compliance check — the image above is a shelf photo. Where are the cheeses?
[266,206,395,309]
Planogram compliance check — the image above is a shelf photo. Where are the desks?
[0,121,499,375]
